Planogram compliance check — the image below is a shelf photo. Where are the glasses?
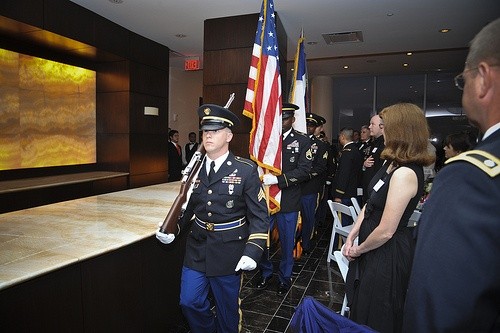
[455,63,500,91]
[379,124,384,129]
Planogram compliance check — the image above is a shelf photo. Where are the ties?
[176,144,181,156]
[208,161,215,182]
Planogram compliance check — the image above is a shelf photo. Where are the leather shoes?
[303,247,309,255]
[276,280,292,296]
[253,276,272,290]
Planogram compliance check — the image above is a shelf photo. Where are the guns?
[159,91,237,239]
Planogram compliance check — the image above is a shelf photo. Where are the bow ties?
[189,142,194,145]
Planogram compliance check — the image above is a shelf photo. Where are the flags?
[242,0,283,213]
[287,27,310,136]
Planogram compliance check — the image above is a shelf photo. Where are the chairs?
[327,197,362,316]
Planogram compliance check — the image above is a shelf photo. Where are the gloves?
[234,255,257,272]
[263,172,279,186]
[155,222,176,245]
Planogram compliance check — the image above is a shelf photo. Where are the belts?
[194,215,247,232]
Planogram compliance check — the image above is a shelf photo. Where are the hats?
[306,112,319,126]
[282,102,299,118]
[318,116,327,125]
[197,104,241,130]
[320,131,325,138]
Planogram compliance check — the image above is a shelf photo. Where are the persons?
[185,132,200,165]
[302,113,471,255]
[257,102,313,296]
[156,103,269,333]
[343,104,438,333]
[403,19,500,333]
[168,128,182,183]
